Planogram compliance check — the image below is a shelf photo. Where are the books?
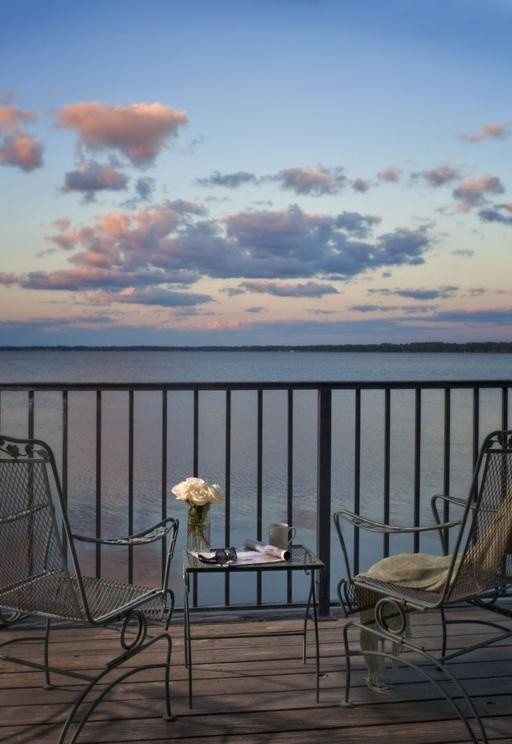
[192,538,289,567]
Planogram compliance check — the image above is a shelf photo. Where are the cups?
[268,522,297,550]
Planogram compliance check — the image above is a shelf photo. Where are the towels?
[353,483,512,694]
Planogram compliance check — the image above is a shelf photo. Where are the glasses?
[198,546,238,564]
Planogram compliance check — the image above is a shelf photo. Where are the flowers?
[170,476,223,546]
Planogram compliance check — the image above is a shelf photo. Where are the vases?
[186,505,209,553]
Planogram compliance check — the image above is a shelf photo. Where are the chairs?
[0,434,178,744]
[332,430,512,744]
